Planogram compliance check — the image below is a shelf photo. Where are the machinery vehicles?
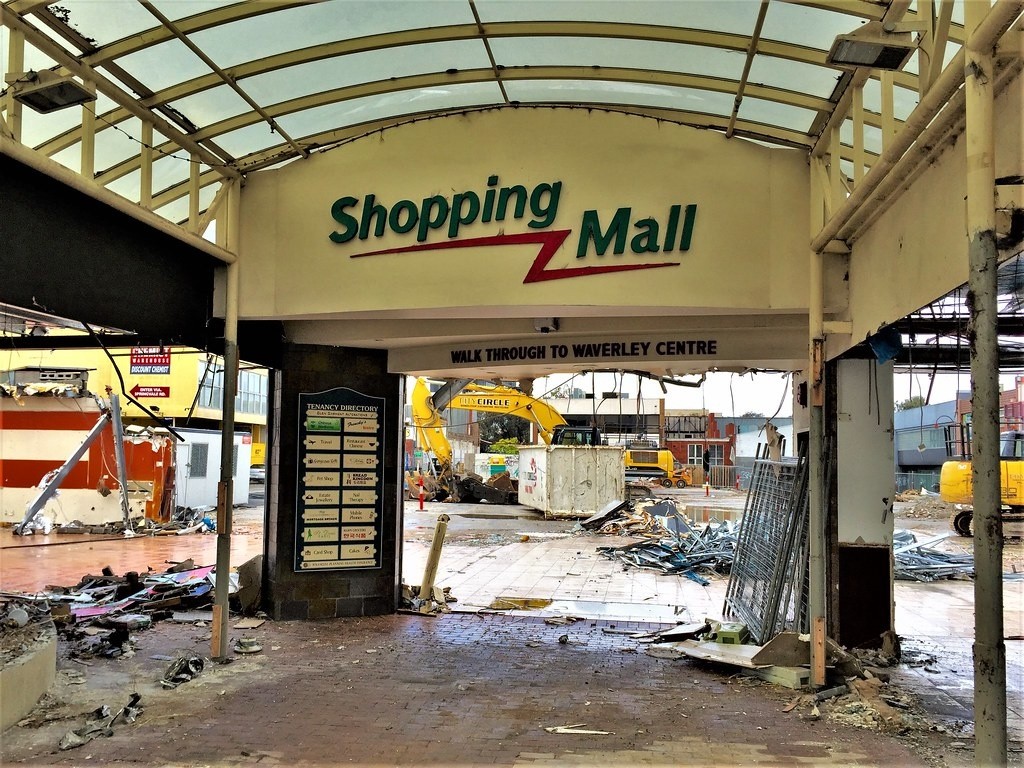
[403,377,652,504]
[940,421,1024,538]
[625,443,692,488]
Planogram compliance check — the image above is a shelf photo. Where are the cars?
[250,464,265,484]
